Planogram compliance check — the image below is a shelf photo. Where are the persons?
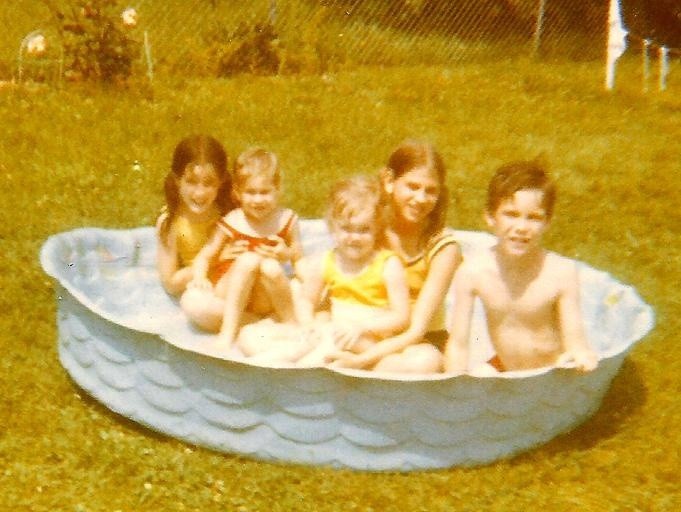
[445,160,599,372]
[237,141,462,373]
[158,134,274,334]
[190,147,304,358]
[236,180,410,364]
